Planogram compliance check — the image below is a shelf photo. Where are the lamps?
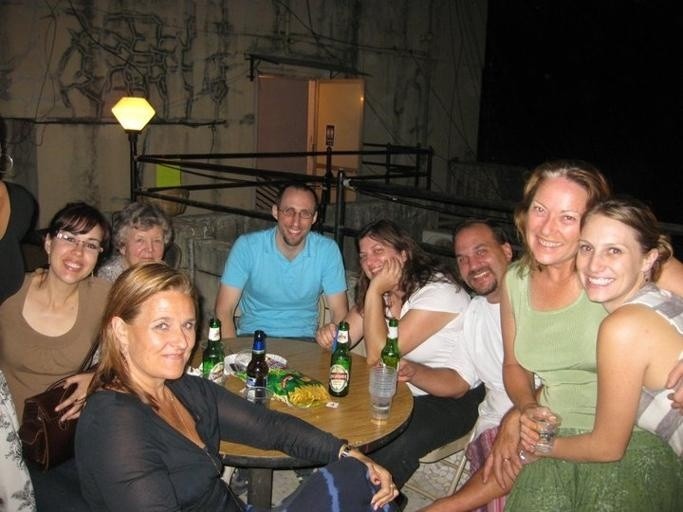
[111,96,156,143]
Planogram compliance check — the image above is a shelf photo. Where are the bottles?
[245,329,270,407]
[201,317,225,386]
[328,319,351,398]
[380,318,401,372]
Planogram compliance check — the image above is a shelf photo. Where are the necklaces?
[148,388,193,442]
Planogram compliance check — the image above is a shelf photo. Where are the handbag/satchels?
[18,364,100,472]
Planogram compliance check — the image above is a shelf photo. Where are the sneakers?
[295,466,318,485]
[229,467,249,496]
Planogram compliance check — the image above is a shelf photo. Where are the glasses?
[55,230,104,255]
[278,206,316,220]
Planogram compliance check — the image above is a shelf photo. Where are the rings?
[502,458,511,462]
[519,450,526,460]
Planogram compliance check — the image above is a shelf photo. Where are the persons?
[0,118,36,512]
[530,198,683,463]
[74,261,401,512]
[315,220,485,512]
[90,201,174,367]
[500,158,683,512]
[0,202,112,512]
[414,385,545,512]
[214,184,349,497]
[376,217,545,512]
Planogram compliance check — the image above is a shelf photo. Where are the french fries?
[287,383,330,406]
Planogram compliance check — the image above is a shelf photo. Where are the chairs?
[402,424,480,501]
[233,294,329,332]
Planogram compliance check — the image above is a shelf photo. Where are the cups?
[241,389,273,410]
[366,365,398,421]
[524,407,562,455]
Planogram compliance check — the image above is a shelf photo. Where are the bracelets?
[339,444,357,458]
